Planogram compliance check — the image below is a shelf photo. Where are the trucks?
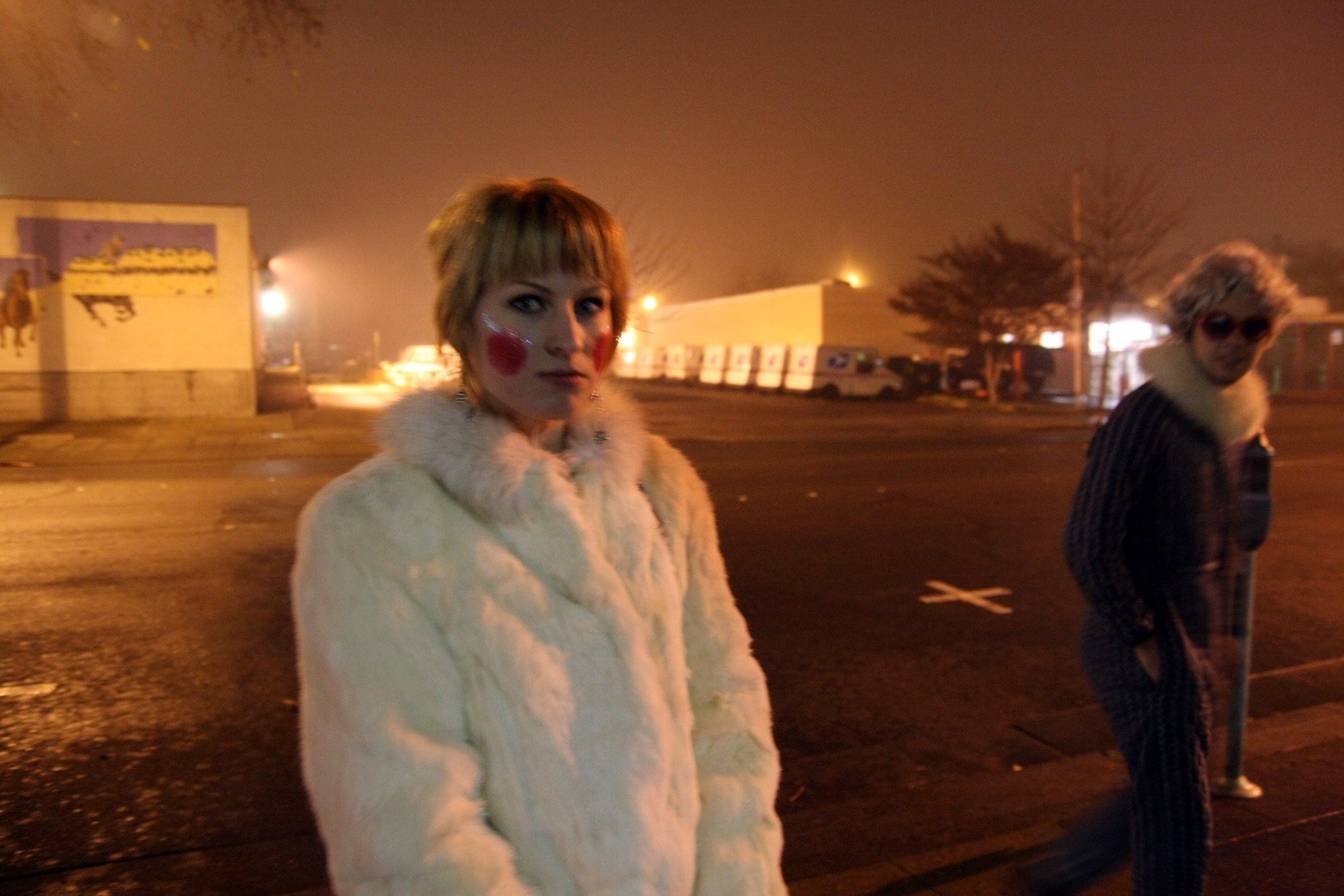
[633,341,909,399]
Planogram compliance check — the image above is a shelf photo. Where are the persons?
[283,174,791,896]
[1063,239,1298,896]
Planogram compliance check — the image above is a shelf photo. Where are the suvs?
[887,354,943,384]
[947,342,1054,396]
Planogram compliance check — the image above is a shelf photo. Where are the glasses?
[1195,309,1276,344]
